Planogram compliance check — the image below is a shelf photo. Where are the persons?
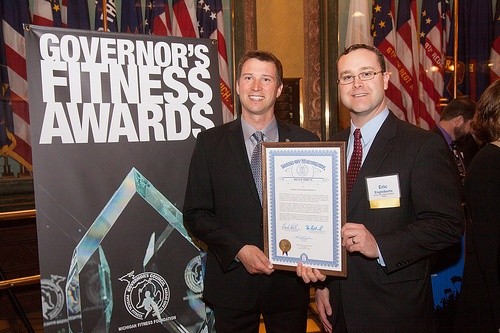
[315,44,466,333]
[183,50,326,333]
[467,78,499,333]
[454,126,488,164]
[432,95,477,199]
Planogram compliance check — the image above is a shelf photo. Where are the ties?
[250,131,264,206]
[450,142,467,187]
[346,128,363,210]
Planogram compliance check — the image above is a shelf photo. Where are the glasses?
[336,70,383,84]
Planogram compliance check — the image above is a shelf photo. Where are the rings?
[352,237,355,244]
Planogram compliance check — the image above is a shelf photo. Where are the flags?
[0,0,233,172]
[346,0,500,131]
